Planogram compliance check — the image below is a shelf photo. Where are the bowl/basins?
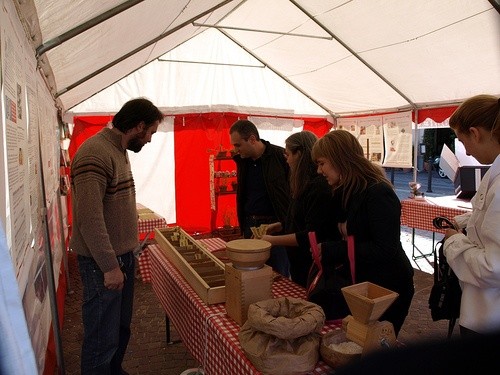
[320,328,364,365]
[226,239,272,270]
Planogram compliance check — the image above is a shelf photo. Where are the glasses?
[283,151,294,159]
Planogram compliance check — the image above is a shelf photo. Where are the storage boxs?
[155,226,226,305]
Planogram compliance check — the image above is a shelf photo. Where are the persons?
[72,98,164,375]
[231,119,415,340]
[442,95,500,334]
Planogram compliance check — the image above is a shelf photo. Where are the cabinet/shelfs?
[213,156,237,194]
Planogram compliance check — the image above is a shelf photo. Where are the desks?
[138,238,342,375]
[400,195,473,259]
[136,201,166,256]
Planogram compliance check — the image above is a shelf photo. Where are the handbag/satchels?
[427,217,468,322]
[306,231,357,321]
